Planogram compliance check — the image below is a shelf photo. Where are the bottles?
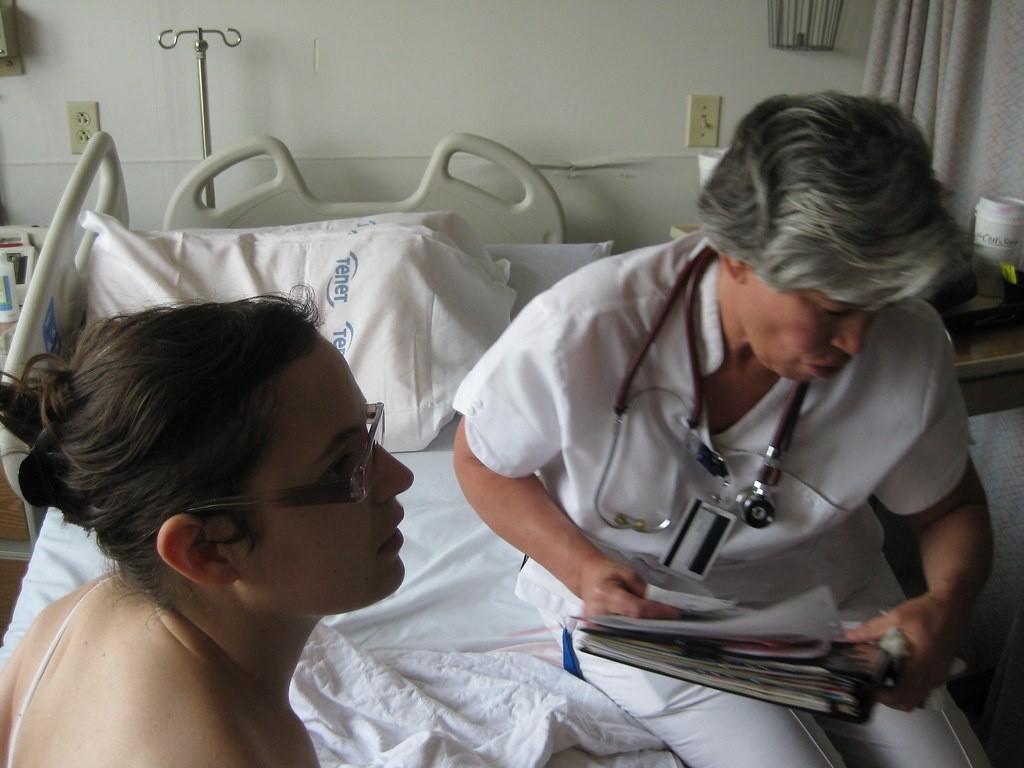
[0,252,19,323]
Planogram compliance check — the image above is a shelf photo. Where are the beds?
[0,133,995,768]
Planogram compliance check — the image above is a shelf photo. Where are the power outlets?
[67,102,100,154]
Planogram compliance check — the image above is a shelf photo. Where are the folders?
[578,625,891,725]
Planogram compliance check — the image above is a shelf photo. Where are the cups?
[973,194,1024,264]
[698,152,724,186]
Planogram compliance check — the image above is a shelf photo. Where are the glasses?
[173,402,386,515]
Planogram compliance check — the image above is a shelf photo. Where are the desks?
[953,324,1024,416]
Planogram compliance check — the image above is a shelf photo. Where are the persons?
[0,296,418,768]
[458,89,994,768]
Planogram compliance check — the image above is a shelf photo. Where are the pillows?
[78,209,518,454]
